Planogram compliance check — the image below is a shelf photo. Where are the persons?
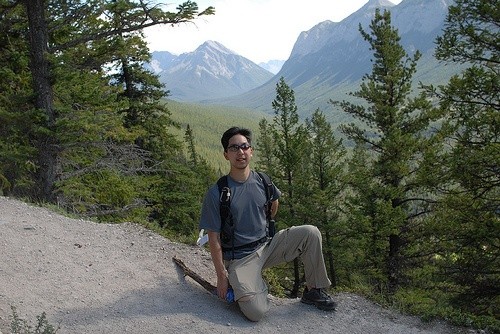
[198,126,337,322]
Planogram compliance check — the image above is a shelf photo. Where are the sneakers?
[300,285,339,312]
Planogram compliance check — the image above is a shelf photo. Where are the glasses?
[226,143,252,152]
